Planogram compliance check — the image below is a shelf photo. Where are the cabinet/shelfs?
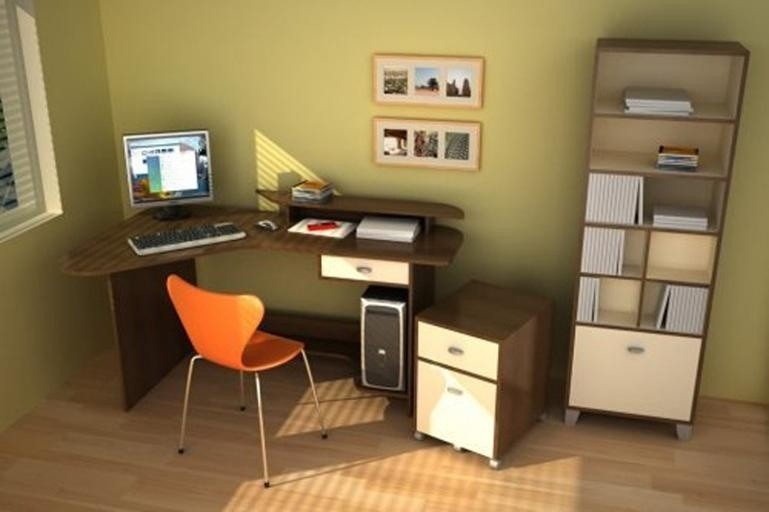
[563,39,751,441]
[413,279,555,468]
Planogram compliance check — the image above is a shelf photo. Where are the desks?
[56,189,465,418]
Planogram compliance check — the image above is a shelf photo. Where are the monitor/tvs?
[122,129,214,221]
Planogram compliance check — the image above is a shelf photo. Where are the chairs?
[165,273,328,488]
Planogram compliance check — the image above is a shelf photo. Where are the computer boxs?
[359,285,409,393]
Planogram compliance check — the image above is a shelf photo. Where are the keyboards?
[127,221,247,256]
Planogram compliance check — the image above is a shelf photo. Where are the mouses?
[256,219,280,232]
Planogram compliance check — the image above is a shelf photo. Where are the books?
[292,179,334,200]
[287,217,357,239]
[355,214,423,244]
[574,85,711,337]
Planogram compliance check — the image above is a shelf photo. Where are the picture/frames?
[373,115,482,171]
[372,54,485,108]
